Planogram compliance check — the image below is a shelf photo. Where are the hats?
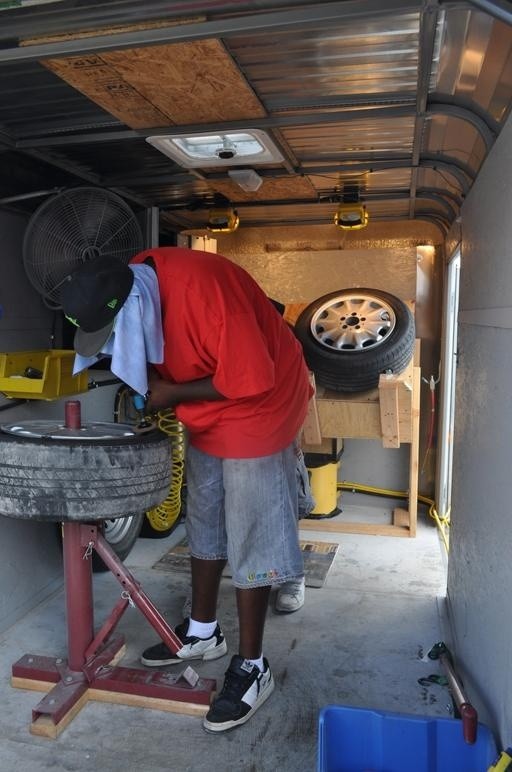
[61,256,134,356]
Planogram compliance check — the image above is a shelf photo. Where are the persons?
[63,246,310,733]
[267,296,317,614]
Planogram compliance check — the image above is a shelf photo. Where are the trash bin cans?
[304,452,342,519]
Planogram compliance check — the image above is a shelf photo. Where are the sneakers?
[276,576,307,613]
[141,617,227,667]
[203,654,275,732]
